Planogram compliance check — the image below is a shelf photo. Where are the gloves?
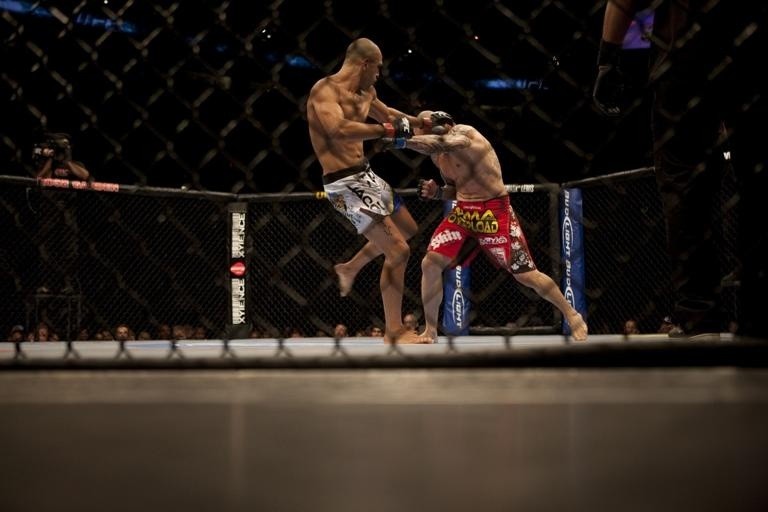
[379,117,415,139]
[588,39,626,121]
[372,137,409,155]
[416,178,444,203]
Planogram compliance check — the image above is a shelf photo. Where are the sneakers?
[666,321,722,341]
[734,320,768,346]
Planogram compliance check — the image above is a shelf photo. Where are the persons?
[25,131,91,295]
[374,110,590,344]
[504,304,637,335]
[305,37,447,344]
[592,0,767,343]
[7,314,426,342]
